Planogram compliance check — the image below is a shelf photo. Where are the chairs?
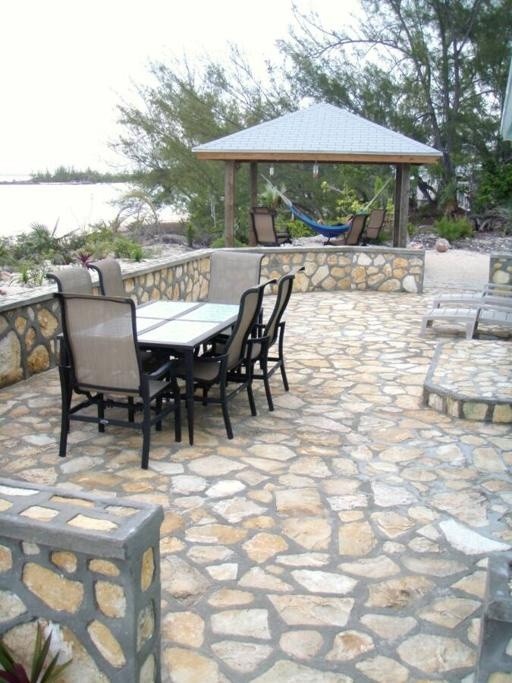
[324,214,369,245]
[44,267,146,422]
[360,209,388,246]
[250,211,288,247]
[207,250,267,303]
[87,257,156,351]
[51,292,181,468]
[203,261,306,411]
[253,207,289,237]
[155,279,277,440]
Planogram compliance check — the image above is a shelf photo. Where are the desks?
[74,297,262,446]
